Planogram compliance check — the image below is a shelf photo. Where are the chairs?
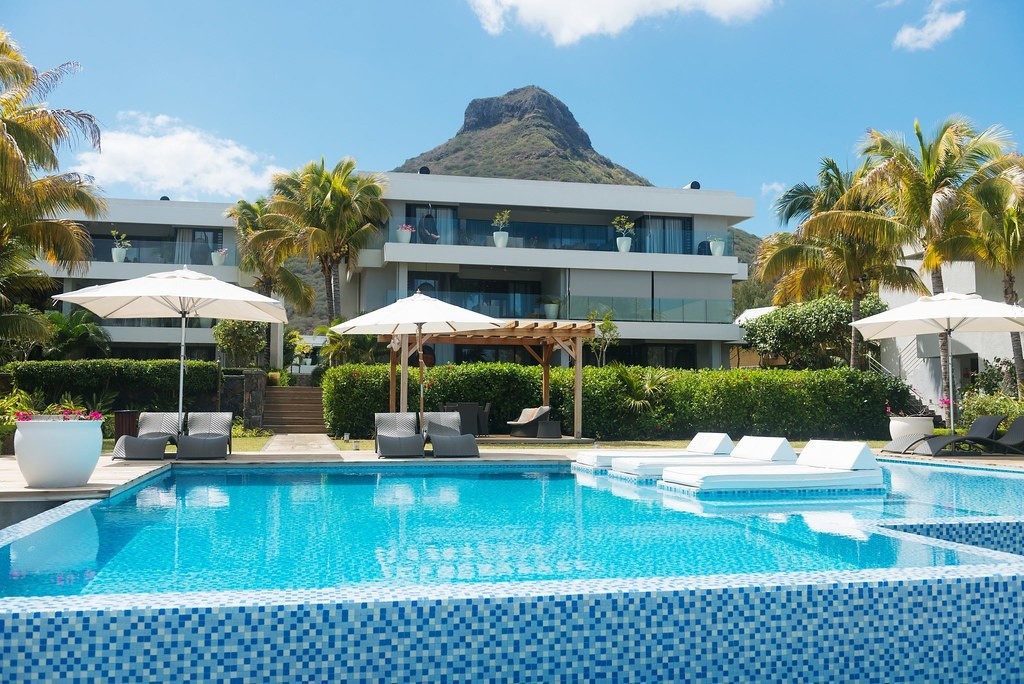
[111,412,186,460]
[439,401,491,437]
[881,415,1009,453]
[423,412,480,457]
[507,406,551,437]
[911,416,1024,457]
[176,412,233,460]
[375,412,425,459]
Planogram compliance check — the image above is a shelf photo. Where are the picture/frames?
[14,421,103,487]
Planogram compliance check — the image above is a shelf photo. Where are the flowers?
[400,224,416,232]
[15,410,102,421]
[886,398,950,416]
[219,247,228,256]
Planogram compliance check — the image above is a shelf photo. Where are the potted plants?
[536,293,559,319]
[491,209,511,247]
[111,230,132,261]
[612,215,635,252]
[710,235,725,256]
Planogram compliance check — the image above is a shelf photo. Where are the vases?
[211,252,225,265]
[397,230,412,242]
[889,417,934,440]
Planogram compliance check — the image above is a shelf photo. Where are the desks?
[537,421,561,437]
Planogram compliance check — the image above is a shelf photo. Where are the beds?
[612,456,796,475]
[662,464,882,487]
[577,450,729,466]
